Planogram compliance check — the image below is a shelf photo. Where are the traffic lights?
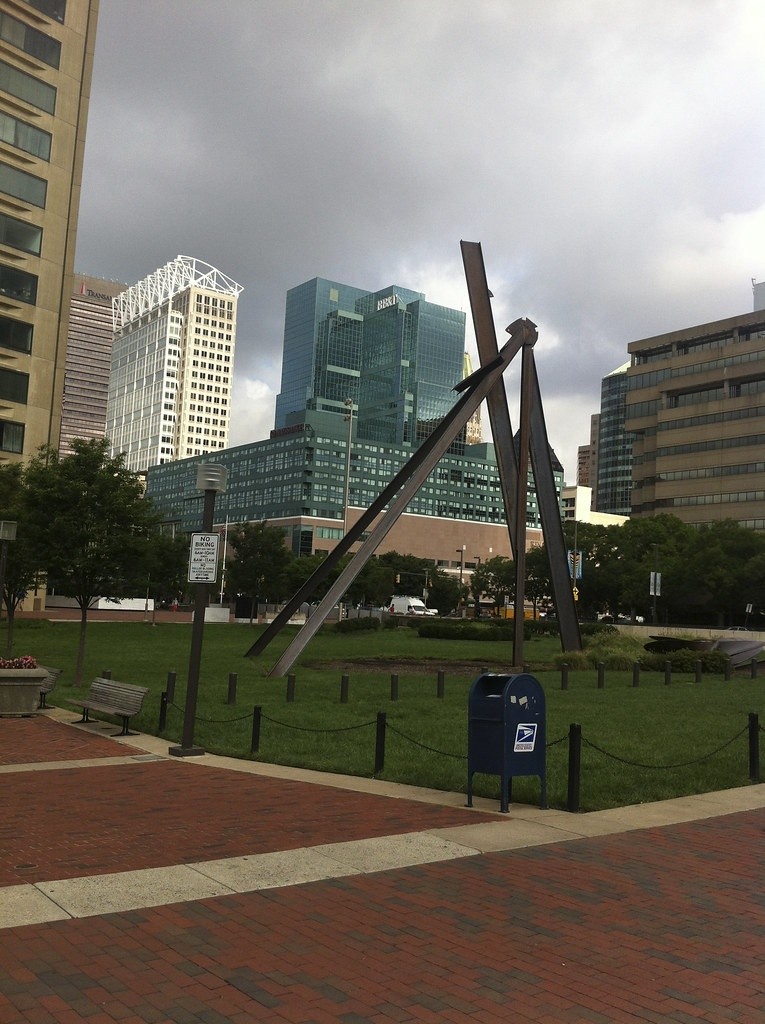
[396,574,401,584]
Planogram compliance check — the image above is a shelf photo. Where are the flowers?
[0,655,36,670]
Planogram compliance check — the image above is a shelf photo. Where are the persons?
[388,604,397,614]
[171,597,179,613]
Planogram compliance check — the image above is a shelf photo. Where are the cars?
[726,625,763,631]
[379,605,391,613]
[596,610,644,624]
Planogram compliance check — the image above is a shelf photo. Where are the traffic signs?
[188,533,220,584]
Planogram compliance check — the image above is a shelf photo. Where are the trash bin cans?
[236,594,259,619]
[539,612,548,623]
[465,670,548,814]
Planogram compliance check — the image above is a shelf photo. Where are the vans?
[389,594,435,616]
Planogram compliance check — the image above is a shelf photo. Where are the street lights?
[167,463,229,757]
[473,556,480,564]
[455,549,463,589]
[341,396,355,538]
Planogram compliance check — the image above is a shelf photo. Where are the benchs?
[64,675,149,737]
[34,664,63,709]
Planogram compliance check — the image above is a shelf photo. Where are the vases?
[0,666,50,716]
